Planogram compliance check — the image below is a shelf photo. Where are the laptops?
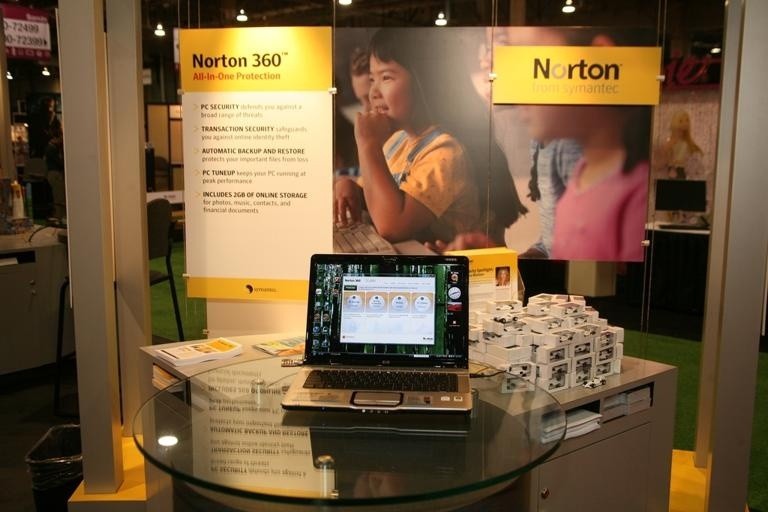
[281,253,473,416]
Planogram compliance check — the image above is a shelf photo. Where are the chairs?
[52,199,189,427]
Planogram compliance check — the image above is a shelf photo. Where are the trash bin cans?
[24,425,84,512]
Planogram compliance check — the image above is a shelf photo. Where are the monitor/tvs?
[654,179,706,213]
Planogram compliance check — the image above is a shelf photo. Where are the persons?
[496,267,509,284]
[33,96,64,162]
[335,27,477,243]
[424,27,644,262]
[657,110,704,165]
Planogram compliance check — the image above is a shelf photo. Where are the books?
[602,386,652,424]
[541,409,602,444]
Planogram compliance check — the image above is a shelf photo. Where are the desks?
[129,354,566,508]
